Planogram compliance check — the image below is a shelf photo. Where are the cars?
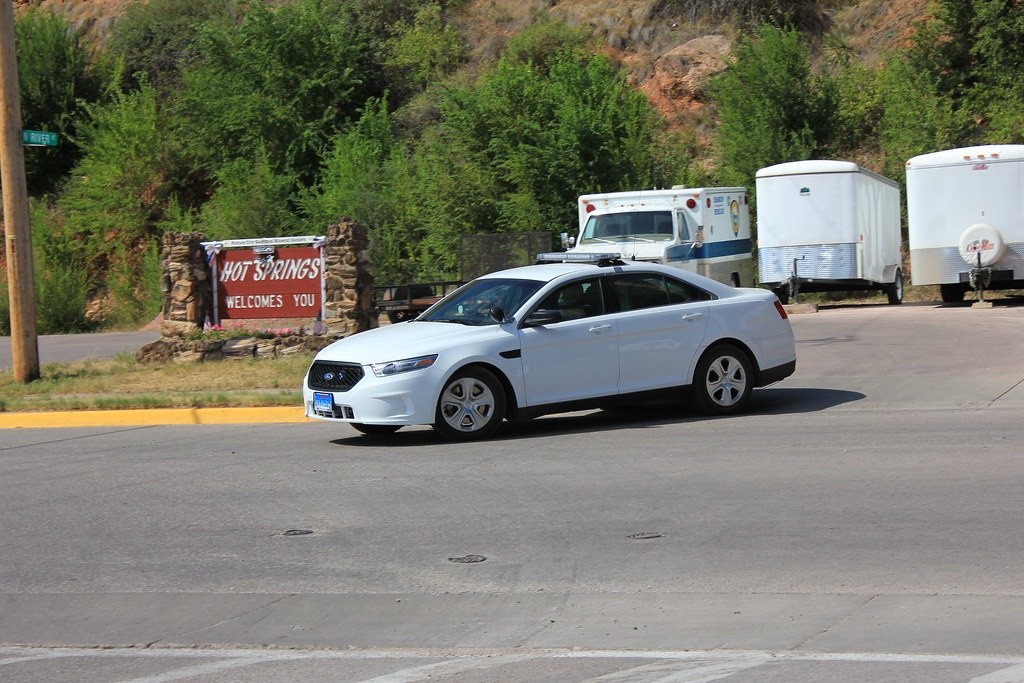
[301,253,795,443]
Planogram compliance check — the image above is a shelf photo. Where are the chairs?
[557,284,586,321]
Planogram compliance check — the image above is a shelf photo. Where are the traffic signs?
[22,130,59,147]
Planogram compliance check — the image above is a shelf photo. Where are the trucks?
[564,184,754,288]
